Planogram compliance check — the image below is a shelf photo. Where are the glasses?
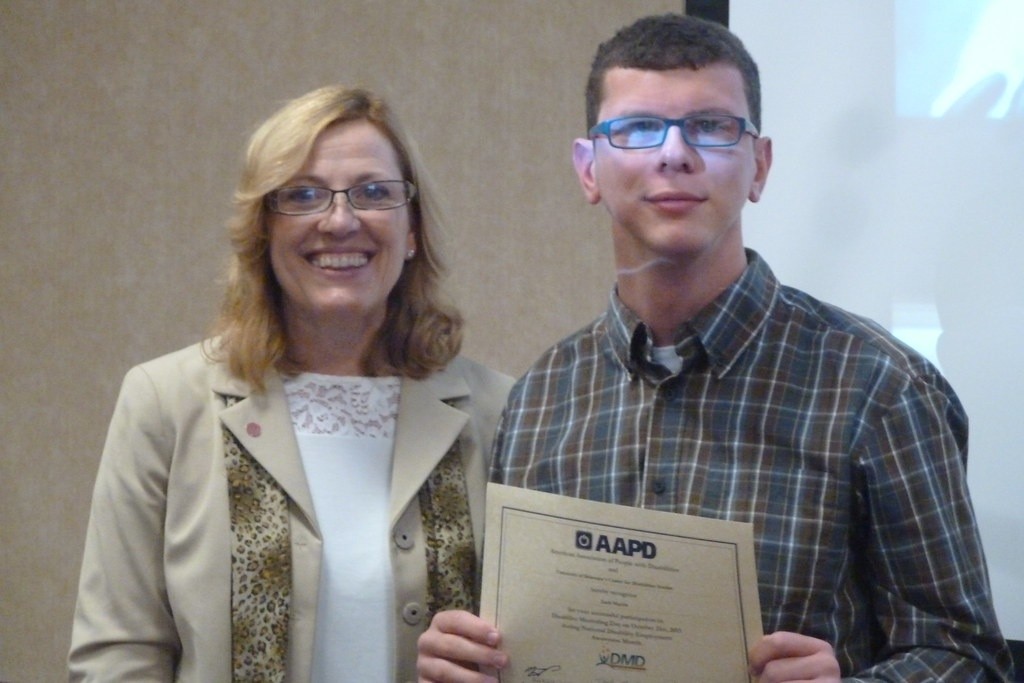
[259,179,417,214]
[587,112,761,149]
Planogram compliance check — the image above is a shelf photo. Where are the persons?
[416,14,1018,683]
[67,88,518,683]
